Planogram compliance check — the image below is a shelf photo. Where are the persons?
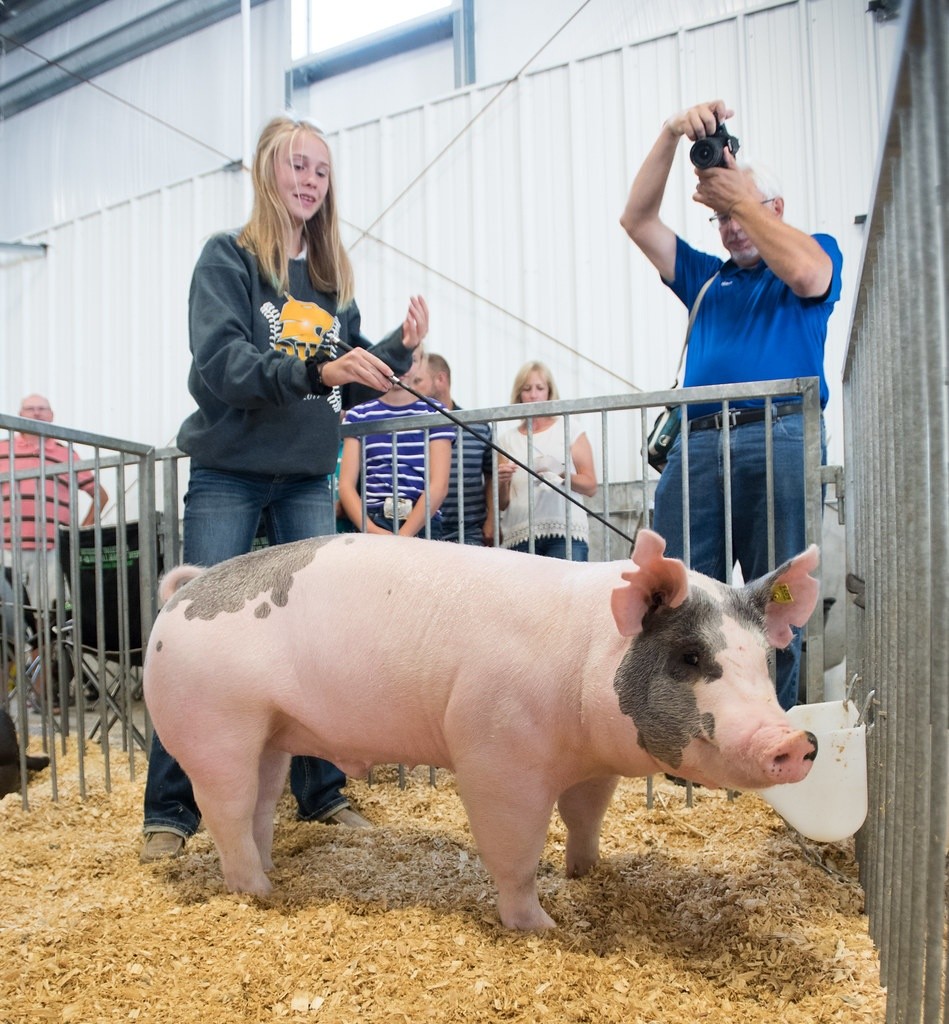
[336,343,458,544]
[425,353,503,548]
[0,393,109,713]
[137,112,428,866]
[620,97,845,713]
[492,360,599,561]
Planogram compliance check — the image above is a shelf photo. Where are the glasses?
[709,198,776,227]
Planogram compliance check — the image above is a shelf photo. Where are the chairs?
[44,512,164,758]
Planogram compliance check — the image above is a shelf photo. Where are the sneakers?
[140,832,183,862]
[326,805,376,829]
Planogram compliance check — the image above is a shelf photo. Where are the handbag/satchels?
[641,404,682,473]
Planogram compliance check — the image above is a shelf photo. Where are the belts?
[690,403,803,432]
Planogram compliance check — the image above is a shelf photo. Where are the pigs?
[141,528,819,935]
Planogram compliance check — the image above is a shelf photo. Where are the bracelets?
[316,358,332,387]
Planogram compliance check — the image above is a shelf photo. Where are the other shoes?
[32,700,60,715]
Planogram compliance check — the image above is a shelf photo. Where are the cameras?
[689,119,740,169]
